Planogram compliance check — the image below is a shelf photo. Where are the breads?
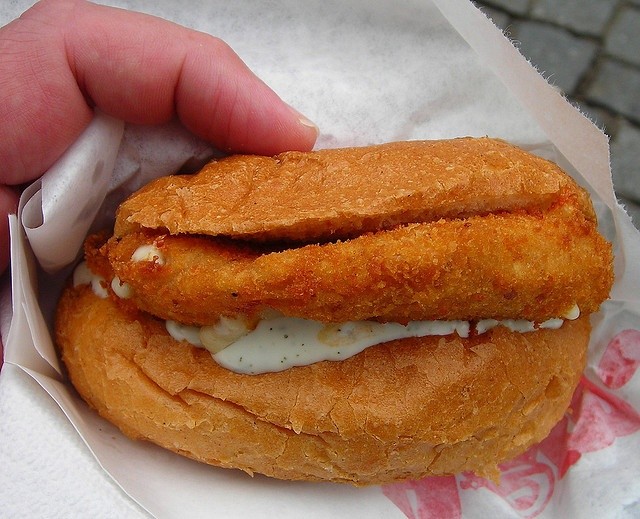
[54,135,616,488]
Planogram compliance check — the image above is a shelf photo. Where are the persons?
[0,0,320,282]
